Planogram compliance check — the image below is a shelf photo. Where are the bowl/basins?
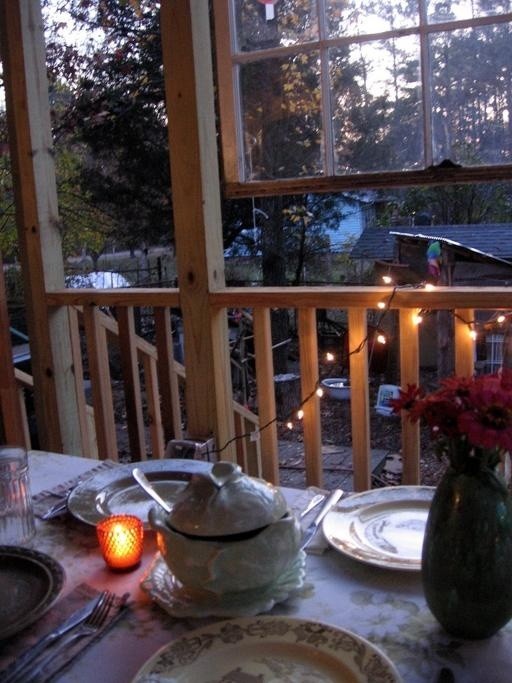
[161,509,302,593]
[322,378,351,400]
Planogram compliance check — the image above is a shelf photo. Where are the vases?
[421,449,512,641]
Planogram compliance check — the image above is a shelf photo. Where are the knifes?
[4,592,105,681]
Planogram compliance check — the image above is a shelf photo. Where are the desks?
[0,446,511,681]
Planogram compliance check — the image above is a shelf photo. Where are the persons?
[477,337,488,376]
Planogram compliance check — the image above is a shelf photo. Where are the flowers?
[391,369,511,494]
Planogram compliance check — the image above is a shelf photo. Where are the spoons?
[303,486,341,557]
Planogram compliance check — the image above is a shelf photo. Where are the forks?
[17,591,115,680]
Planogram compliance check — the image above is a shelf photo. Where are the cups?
[0,447,37,546]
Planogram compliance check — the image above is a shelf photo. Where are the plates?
[147,550,307,620]
[65,459,214,531]
[323,483,436,571]
[1,546,66,641]
[126,616,402,683]
